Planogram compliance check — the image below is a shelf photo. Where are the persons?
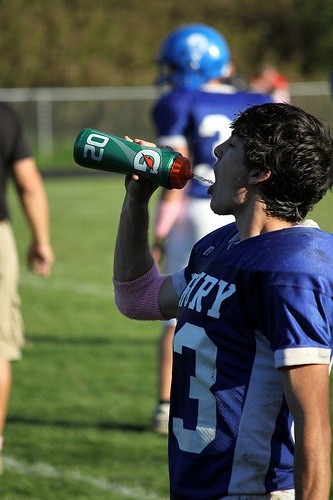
[113,103,332,500]
[221,61,291,104]
[147,22,278,435]
[0,101,56,450]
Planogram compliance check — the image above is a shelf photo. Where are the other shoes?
[154,402,170,433]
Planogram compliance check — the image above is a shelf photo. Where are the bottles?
[72,127,194,191]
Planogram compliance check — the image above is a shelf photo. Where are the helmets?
[153,23,231,97]
